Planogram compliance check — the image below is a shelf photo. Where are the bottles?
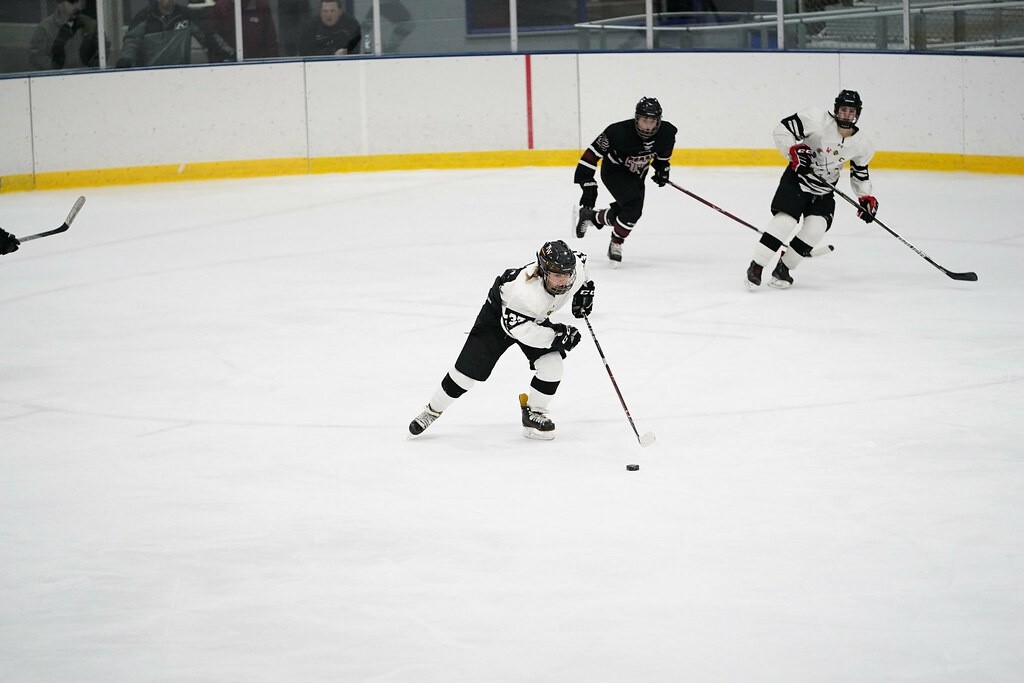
[363,35,372,54]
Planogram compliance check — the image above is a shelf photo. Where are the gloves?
[0,229,20,255]
[572,281,595,318]
[580,181,598,210]
[116,58,132,68]
[790,144,816,174]
[551,323,581,359]
[651,165,670,187]
[857,195,878,223]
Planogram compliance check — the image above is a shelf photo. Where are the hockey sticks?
[811,169,980,282]
[18,195,87,244]
[581,307,656,445]
[664,180,835,258]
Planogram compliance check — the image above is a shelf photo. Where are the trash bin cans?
[752,15,778,49]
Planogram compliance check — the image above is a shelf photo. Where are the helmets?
[536,240,576,295]
[834,90,862,130]
[635,97,662,139]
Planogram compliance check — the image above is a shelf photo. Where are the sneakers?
[607,231,624,269]
[745,260,764,291]
[406,403,443,440]
[519,393,555,440]
[572,205,604,240]
[767,251,794,289]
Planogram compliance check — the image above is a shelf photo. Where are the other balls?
[626,464,640,471]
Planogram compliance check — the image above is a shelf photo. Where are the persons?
[30,0,362,71]
[406,240,596,440]
[574,97,678,269]
[0,228,20,255]
[747,89,880,292]
